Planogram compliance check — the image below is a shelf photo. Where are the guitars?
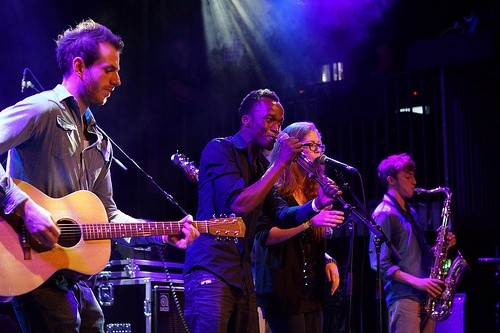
[0,178,246,303]
[171,150,200,181]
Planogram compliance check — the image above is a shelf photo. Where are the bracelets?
[303,221,309,228]
[312,198,321,212]
[326,258,337,264]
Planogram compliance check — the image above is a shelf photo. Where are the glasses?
[301,143,326,153]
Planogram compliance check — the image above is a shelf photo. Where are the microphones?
[318,155,357,172]
[277,132,314,167]
[21,72,26,93]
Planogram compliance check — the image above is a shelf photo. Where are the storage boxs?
[90,278,184,333]
[434,292,467,333]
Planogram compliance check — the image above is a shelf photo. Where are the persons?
[253,121,345,333]
[182,89,343,333]
[368,153,457,333]
[0,20,201,333]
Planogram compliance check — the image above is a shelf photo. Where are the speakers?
[433,291,497,333]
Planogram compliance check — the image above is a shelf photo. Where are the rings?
[37,241,42,244]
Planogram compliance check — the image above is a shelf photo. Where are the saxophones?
[413,185,469,320]
